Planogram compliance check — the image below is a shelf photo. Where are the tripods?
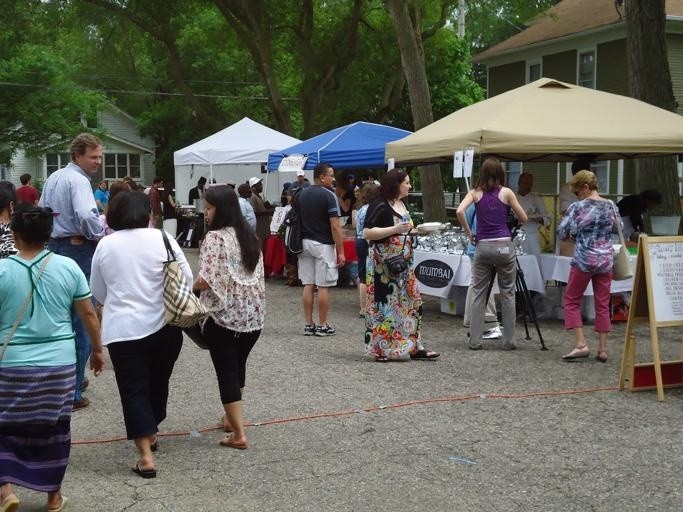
[485,227,549,351]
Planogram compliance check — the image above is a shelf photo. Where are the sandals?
[595,351,610,362]
[560,344,591,359]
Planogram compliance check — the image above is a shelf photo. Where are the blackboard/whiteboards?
[642,235,683,327]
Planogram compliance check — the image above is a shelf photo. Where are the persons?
[616,189,662,241]
[189,175,275,240]
[558,159,591,257]
[513,171,548,280]
[89,189,195,479]
[361,167,440,363]
[463,201,503,339]
[1,201,107,511]
[279,163,381,338]
[454,157,529,351]
[558,169,624,362]
[192,185,268,450]
[94,175,177,240]
[0,180,49,259]
[16,173,39,206]
[36,132,105,414]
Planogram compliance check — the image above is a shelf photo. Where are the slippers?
[410,348,441,359]
[220,414,235,432]
[132,460,157,479]
[219,432,249,450]
[0,495,20,512]
[46,494,68,512]
[150,435,159,452]
[375,353,389,362]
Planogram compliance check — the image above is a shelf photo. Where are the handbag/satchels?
[157,226,210,331]
[606,199,633,281]
[282,186,306,255]
[181,288,214,351]
[384,253,408,275]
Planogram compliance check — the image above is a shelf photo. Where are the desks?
[415,252,638,322]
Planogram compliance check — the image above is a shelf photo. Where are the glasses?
[574,186,586,196]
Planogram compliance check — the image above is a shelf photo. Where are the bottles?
[401,210,411,234]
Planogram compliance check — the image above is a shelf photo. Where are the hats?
[227,179,236,186]
[296,169,305,177]
[248,176,264,188]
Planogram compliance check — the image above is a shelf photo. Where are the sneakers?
[315,323,336,336]
[480,326,504,339]
[80,375,90,392]
[303,322,316,336]
[71,396,90,409]
